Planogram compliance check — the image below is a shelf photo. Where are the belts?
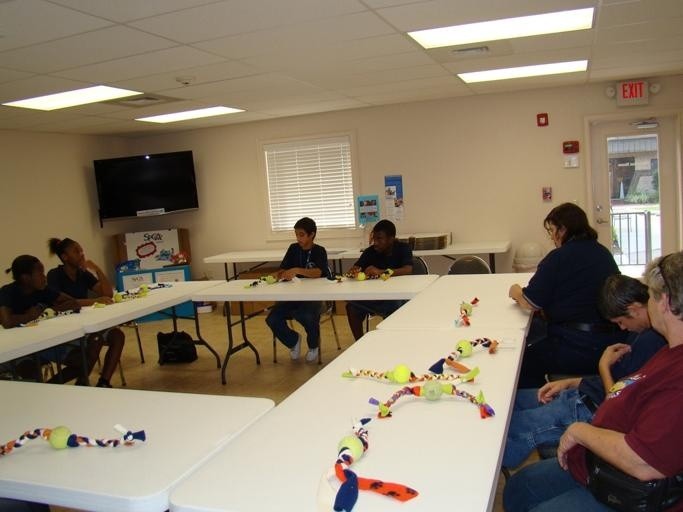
[577,390,598,417]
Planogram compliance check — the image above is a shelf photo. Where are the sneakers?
[305,347,318,362]
[289,334,301,360]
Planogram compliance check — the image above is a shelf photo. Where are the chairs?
[264,264,342,365]
[97,319,146,385]
[366,257,428,332]
[447,255,492,274]
[369,235,447,250]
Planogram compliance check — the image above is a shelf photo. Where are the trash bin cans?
[512,243,543,273]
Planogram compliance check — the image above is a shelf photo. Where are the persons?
[265,218,328,362]
[45,237,124,387]
[0,254,87,383]
[500,275,667,469]
[509,203,621,388]
[504,251,683,510]
[345,219,412,341]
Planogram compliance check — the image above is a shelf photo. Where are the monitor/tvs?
[93,150,199,219]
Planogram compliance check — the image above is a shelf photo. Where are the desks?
[0,379,275,512]
[375,272,536,337]
[192,274,439,387]
[0,310,90,387]
[80,280,228,386]
[203,249,361,280]
[169,329,526,512]
[341,240,510,274]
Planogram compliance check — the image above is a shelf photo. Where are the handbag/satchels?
[157,331,197,365]
[584,450,682,510]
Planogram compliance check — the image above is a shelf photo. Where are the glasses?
[658,255,672,310]
